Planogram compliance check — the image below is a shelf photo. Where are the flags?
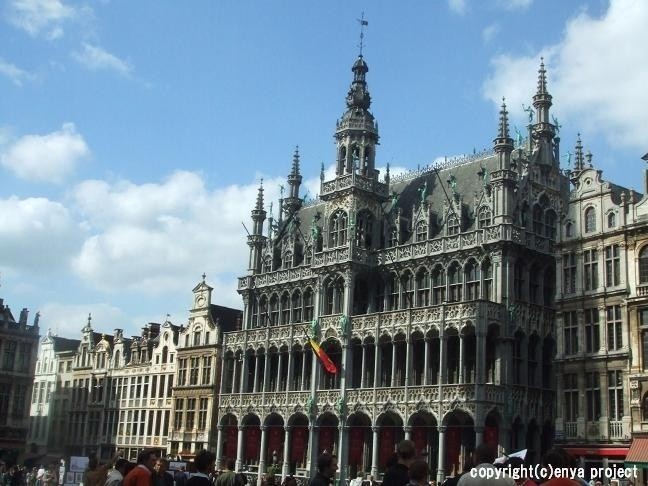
[309,338,337,376]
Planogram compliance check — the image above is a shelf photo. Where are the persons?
[0,436,637,485]
